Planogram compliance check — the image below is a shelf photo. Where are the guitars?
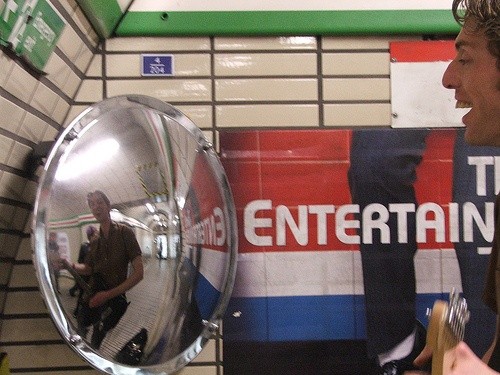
[428,288,471,374]
[59,259,129,330]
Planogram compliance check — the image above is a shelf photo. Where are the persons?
[405,0,500,375]
[48,232,62,295]
[70,226,98,320]
[53,190,144,352]
